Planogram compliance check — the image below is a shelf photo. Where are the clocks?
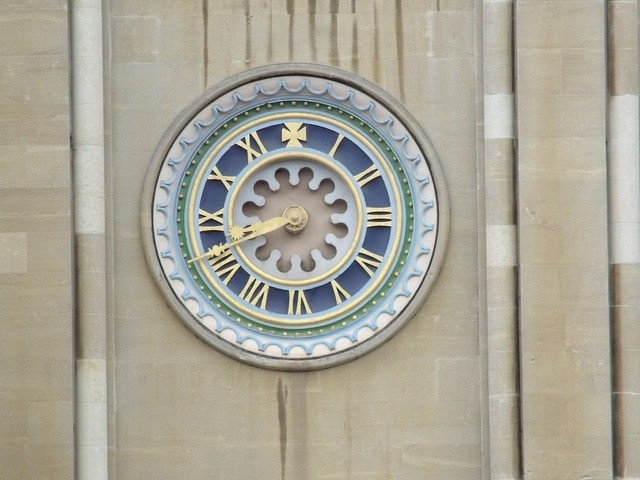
[137,62,452,372]
